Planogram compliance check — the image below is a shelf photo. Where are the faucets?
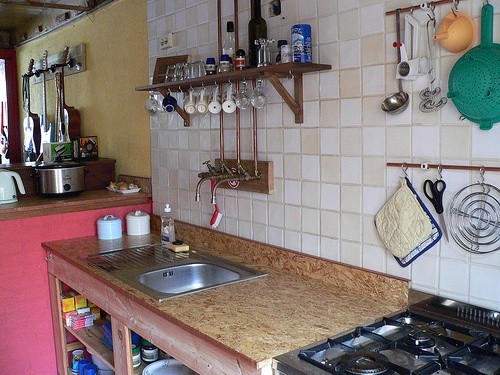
[194,174,221,203]
[210,175,246,205]
[134,262,269,304]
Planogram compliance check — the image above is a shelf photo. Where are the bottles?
[218,54,234,74]
[97,214,122,239]
[222,20,237,58]
[235,49,248,71]
[248,0,267,68]
[126,211,150,235]
[275,39,294,65]
[160,204,175,244]
[204,57,216,75]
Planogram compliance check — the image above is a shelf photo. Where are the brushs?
[210,179,222,229]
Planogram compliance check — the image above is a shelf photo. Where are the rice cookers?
[29,156,85,200]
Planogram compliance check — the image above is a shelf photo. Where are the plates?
[142,359,199,375]
[107,186,141,193]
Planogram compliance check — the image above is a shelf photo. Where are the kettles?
[0,168,26,205]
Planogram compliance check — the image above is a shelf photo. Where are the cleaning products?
[160,203,175,246]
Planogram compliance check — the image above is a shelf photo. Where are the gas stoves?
[270,296,500,375]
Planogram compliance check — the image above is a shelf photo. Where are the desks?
[0,156,116,194]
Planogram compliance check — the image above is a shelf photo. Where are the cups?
[291,24,312,64]
[222,88,236,114]
[164,60,207,82]
[183,91,198,114]
[208,87,223,114]
[70,350,85,375]
[144,95,159,114]
[196,90,209,113]
[251,80,268,109]
[161,92,177,113]
[233,83,252,110]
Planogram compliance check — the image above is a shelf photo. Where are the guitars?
[21,59,40,156]
[56,45,82,142]
[39,49,54,154]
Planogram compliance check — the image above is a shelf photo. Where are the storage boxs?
[61,292,101,329]
[43,143,74,162]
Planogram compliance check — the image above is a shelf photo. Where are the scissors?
[424,179,450,243]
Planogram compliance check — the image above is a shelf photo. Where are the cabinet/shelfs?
[47,251,261,375]
